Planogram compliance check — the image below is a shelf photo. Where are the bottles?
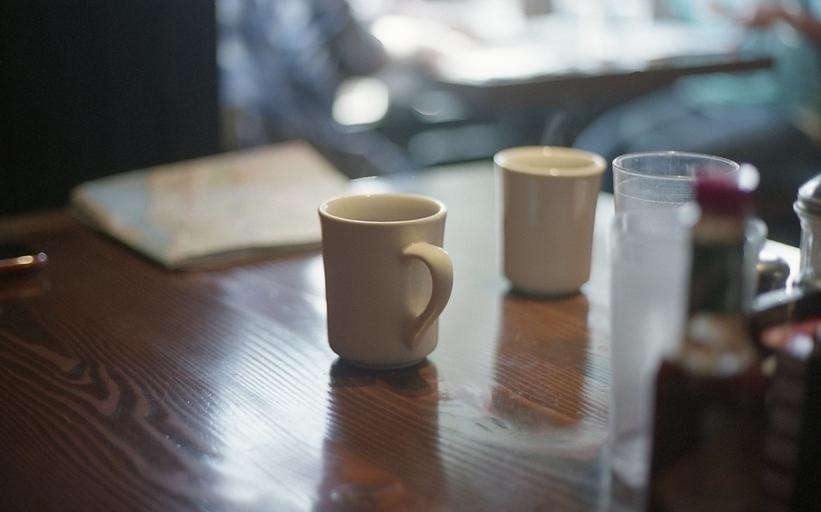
[793,173,821,289]
[641,179,777,512]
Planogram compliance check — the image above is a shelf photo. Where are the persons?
[573,0,821,197]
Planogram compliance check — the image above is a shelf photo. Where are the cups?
[493,142,608,298]
[324,353,447,508]
[316,190,455,372]
[493,288,593,428]
[610,150,743,211]
[606,208,768,490]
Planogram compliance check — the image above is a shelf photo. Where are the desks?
[3,158,818,511]
